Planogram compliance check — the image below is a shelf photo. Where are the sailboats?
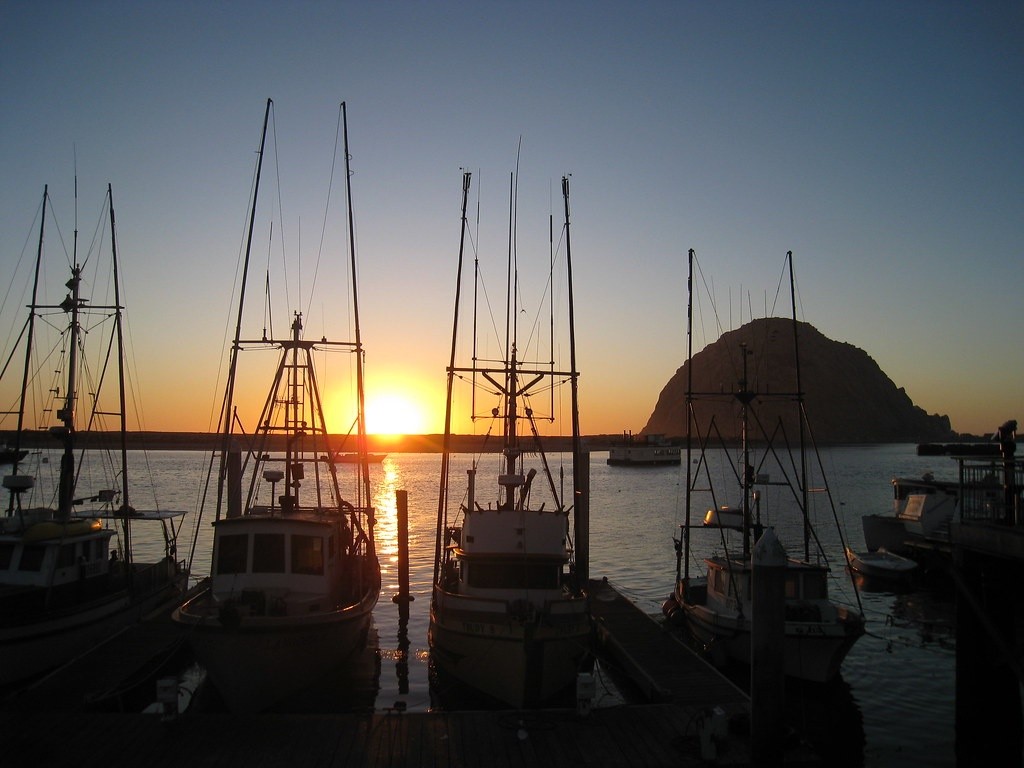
[0,147,190,723]
[172,98,381,685]
[661,248,865,684]
[428,132,613,704]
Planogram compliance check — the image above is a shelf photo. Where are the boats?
[846,546,921,584]
[607,430,681,464]
[861,471,991,552]
[263,453,386,464]
